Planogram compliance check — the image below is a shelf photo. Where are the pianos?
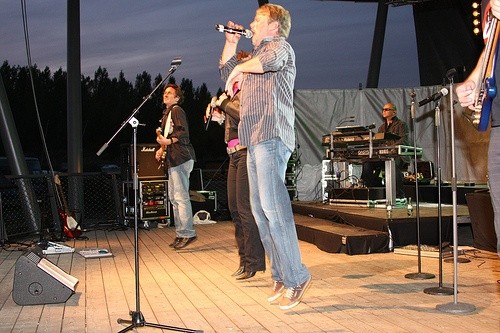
[321,130,423,208]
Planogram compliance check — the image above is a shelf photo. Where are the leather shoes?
[175,235,197,249]
[232,266,245,277]
[169,237,184,247]
[236,270,256,280]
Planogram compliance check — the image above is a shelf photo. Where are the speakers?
[131,143,168,180]
[12,249,79,305]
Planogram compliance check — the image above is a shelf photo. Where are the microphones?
[337,116,355,126]
[416,88,449,107]
[215,25,254,39]
[169,56,182,75]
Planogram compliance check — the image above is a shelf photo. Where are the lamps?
[12,251,78,305]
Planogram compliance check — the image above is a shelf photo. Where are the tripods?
[96,71,205,333]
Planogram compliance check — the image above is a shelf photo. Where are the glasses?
[382,108,395,111]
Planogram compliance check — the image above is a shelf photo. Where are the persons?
[360,103,410,188]
[456,0,500,287]
[205,3,313,310]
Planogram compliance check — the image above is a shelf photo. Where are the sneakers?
[267,280,288,303]
[279,275,312,310]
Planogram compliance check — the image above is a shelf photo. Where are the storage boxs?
[190,191,217,212]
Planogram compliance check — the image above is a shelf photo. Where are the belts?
[226,144,247,154]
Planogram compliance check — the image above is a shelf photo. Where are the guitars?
[155,127,166,175]
[54,174,82,239]
[471,12,500,131]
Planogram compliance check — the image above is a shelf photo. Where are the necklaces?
[154,83,198,249]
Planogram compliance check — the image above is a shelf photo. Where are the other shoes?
[359,178,366,184]
